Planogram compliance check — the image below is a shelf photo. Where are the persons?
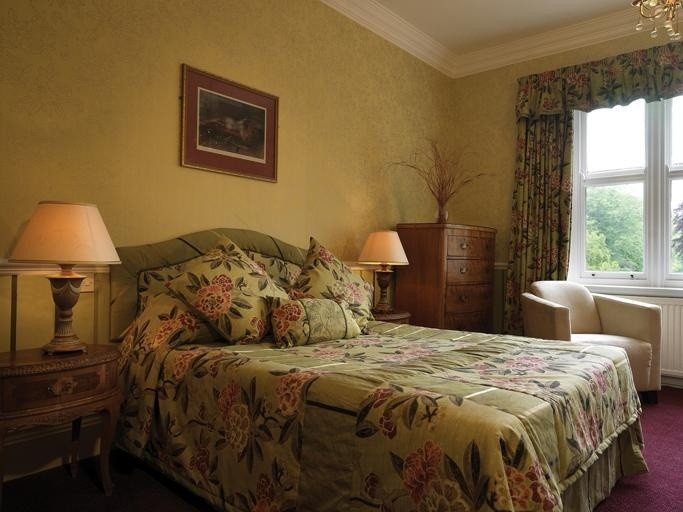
[199,115,259,132]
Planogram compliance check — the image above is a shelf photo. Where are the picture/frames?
[180,64,279,184]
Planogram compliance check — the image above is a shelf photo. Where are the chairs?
[521,280,662,404]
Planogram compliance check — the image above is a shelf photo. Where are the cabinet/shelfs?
[396,224,499,328]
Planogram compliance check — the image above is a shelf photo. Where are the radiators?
[601,293,683,378]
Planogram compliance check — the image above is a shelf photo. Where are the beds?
[92,227,650,512]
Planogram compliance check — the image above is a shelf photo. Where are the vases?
[438,205,449,224]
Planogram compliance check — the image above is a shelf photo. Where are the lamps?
[358,231,409,314]
[8,201,122,355]
[631,0,683,41]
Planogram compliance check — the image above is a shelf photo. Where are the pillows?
[269,297,361,349]
[165,236,291,344]
[291,237,374,321]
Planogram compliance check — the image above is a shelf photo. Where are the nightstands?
[0,344,122,512]
[374,310,411,324]
